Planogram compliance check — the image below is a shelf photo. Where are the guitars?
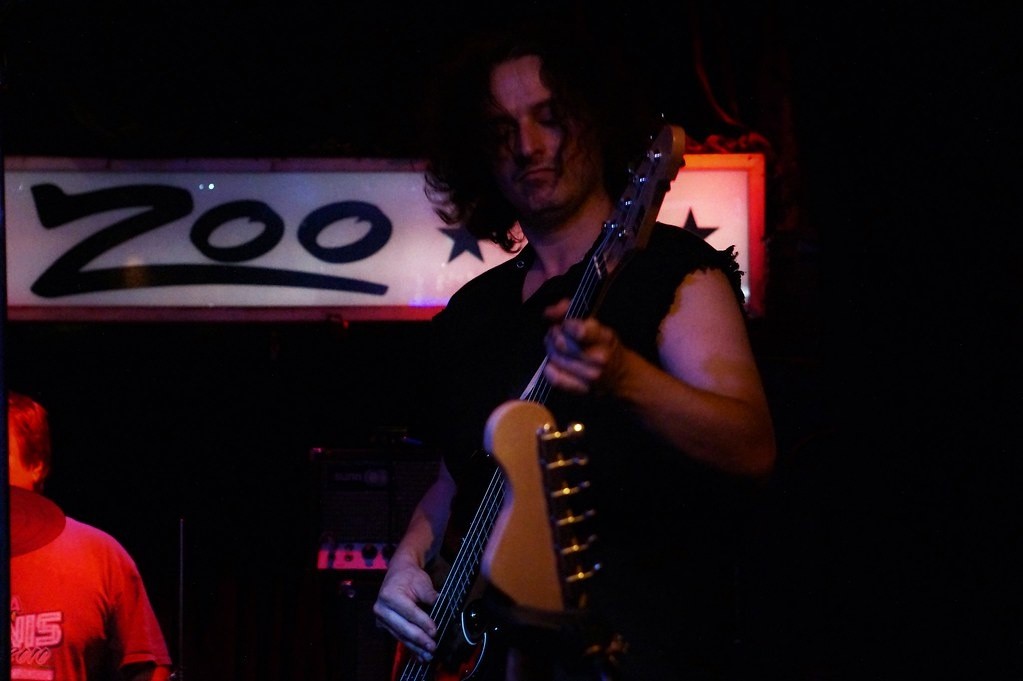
[388,125,684,680]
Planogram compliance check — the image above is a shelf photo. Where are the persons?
[375,18,781,681]
[10,392,173,681]
